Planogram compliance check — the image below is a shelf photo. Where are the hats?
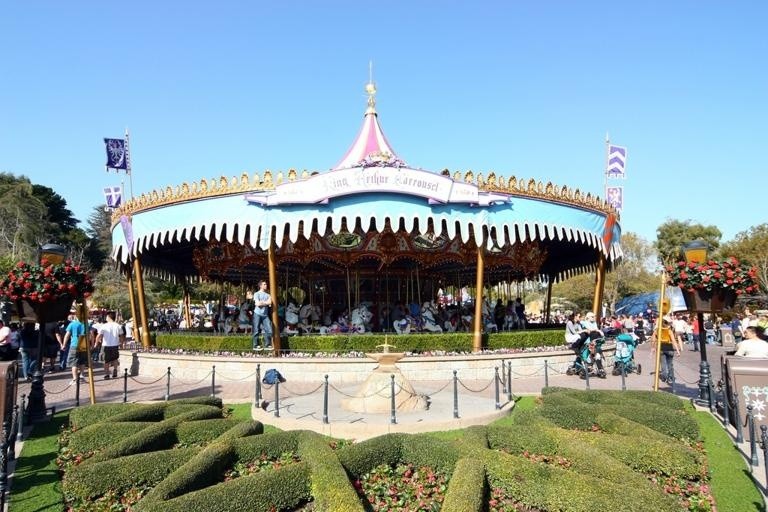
[586,312,594,317]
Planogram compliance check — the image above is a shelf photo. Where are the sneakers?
[253,345,273,351]
[103,369,117,380]
[69,376,86,385]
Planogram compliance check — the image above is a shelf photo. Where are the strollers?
[566,334,641,379]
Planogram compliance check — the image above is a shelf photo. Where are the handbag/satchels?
[262,369,286,384]
[78,334,91,352]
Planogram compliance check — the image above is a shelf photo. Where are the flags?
[607,145,626,175]
[104,187,122,210]
[105,136,128,170]
[607,186,623,209]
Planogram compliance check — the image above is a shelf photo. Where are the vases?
[24,295,74,321]
[681,285,739,316]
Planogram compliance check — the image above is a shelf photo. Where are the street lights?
[24,241,66,419]
[681,240,717,409]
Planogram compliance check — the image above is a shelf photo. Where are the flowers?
[665,255,760,294]
[0,258,97,303]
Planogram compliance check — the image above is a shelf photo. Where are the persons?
[412,296,528,331]
[398,301,410,315]
[0,306,207,385]
[251,279,273,352]
[649,308,768,384]
[524,310,657,376]
